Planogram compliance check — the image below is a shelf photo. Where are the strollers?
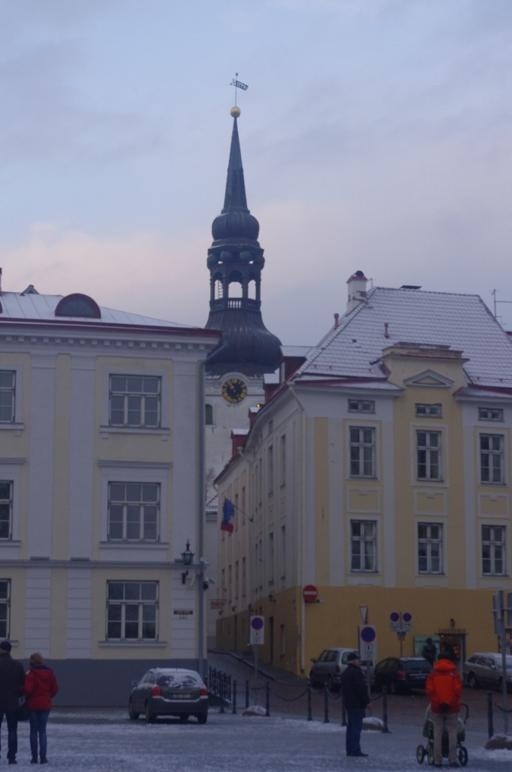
[417,703,469,765]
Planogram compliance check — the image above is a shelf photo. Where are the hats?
[346,653,362,661]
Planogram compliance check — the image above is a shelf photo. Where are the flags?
[220,497,235,535]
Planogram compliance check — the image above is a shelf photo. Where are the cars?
[463,652,512,694]
[375,656,432,694]
[129,667,208,723]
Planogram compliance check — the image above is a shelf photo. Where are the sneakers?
[346,750,369,758]
[8,759,18,765]
[31,756,48,764]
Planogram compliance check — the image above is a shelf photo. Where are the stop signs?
[303,584,319,602]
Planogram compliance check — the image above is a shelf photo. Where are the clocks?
[222,377,247,404]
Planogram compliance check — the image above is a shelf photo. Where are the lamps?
[182,541,195,584]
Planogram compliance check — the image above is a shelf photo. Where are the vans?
[309,647,373,692]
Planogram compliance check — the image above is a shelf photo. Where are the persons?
[21,651,60,763]
[421,636,437,661]
[1,639,27,763]
[426,650,463,766]
[341,652,369,755]
